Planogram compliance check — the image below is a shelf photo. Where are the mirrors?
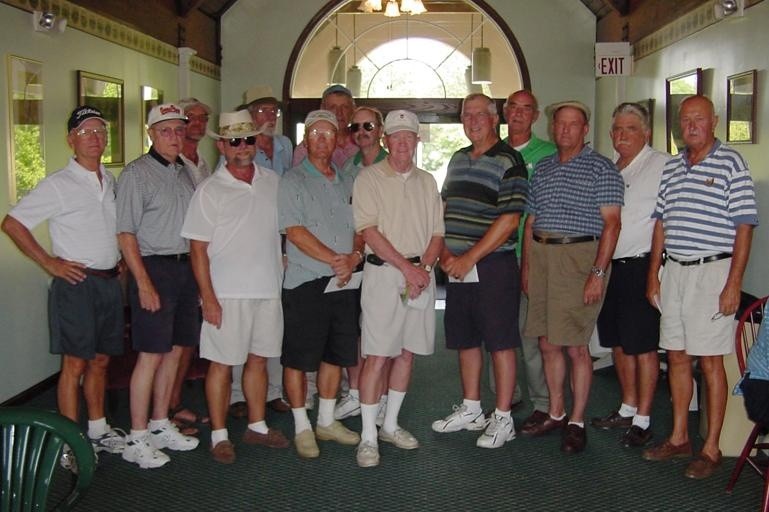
[665,68,701,155]
[141,85,164,155]
[77,70,125,168]
[636,98,653,147]
[726,69,757,145]
[5,54,48,206]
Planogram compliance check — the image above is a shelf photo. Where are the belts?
[141,254,188,264]
[363,257,422,264]
[611,253,652,266]
[79,266,121,280]
[533,233,600,244]
[664,250,733,266]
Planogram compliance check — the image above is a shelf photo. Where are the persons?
[175,98,212,193]
[332,107,395,429]
[353,109,445,466]
[431,94,529,449]
[292,85,361,174]
[179,109,291,462]
[213,84,294,179]
[590,104,675,449]
[277,110,366,459]
[485,90,551,429]
[732,297,769,468]
[518,100,625,452]
[642,95,759,479]
[116,103,201,468]
[0,107,125,474]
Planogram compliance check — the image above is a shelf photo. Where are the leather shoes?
[485,400,523,418]
[242,428,290,449]
[685,449,723,481]
[208,440,235,464]
[560,424,586,454]
[593,411,633,431]
[520,410,569,439]
[642,439,693,461]
[619,426,653,448]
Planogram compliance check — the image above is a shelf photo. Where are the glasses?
[348,122,379,132]
[222,136,257,146]
[72,127,107,137]
[462,112,494,120]
[185,113,208,122]
[151,127,187,136]
[305,128,336,138]
[252,107,279,114]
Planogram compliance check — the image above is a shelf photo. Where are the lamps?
[327,12,345,84]
[357,0,428,17]
[472,14,492,85]
[465,14,483,94]
[346,14,361,97]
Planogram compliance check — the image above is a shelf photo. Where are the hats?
[323,85,351,101]
[177,98,211,115]
[305,110,339,131]
[236,85,283,112]
[68,106,106,135]
[148,103,188,128]
[206,109,269,140]
[383,110,419,135]
[550,101,590,122]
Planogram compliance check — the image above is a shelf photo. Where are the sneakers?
[294,429,320,457]
[477,413,515,449]
[317,421,360,445]
[432,405,485,433]
[265,398,291,412]
[169,417,198,436]
[148,421,199,451]
[229,402,250,418]
[356,438,380,466]
[334,393,361,420]
[122,435,171,468]
[86,425,125,454]
[169,404,210,423]
[60,449,98,477]
[376,399,387,426]
[304,396,314,411]
[379,427,418,449]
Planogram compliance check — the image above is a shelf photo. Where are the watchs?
[352,249,366,264]
[419,263,432,273]
[591,265,606,279]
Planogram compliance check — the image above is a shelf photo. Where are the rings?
[344,281,348,284]
[420,284,424,288]
[455,275,460,279]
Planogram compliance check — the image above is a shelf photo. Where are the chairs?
[0,408,97,512]
[727,295,769,512]
